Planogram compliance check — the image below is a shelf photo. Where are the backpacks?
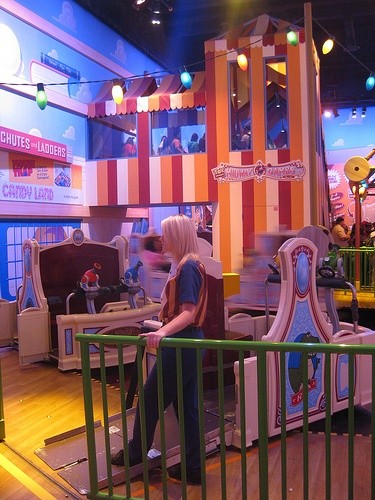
[246,133,251,150]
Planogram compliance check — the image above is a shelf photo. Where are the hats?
[362,221,368,224]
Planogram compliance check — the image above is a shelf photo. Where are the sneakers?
[167,467,183,482]
[111,450,124,466]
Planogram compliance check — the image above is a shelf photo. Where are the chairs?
[18,229,161,371]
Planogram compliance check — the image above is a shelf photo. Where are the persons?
[111,215,208,483]
[331,217,375,285]
